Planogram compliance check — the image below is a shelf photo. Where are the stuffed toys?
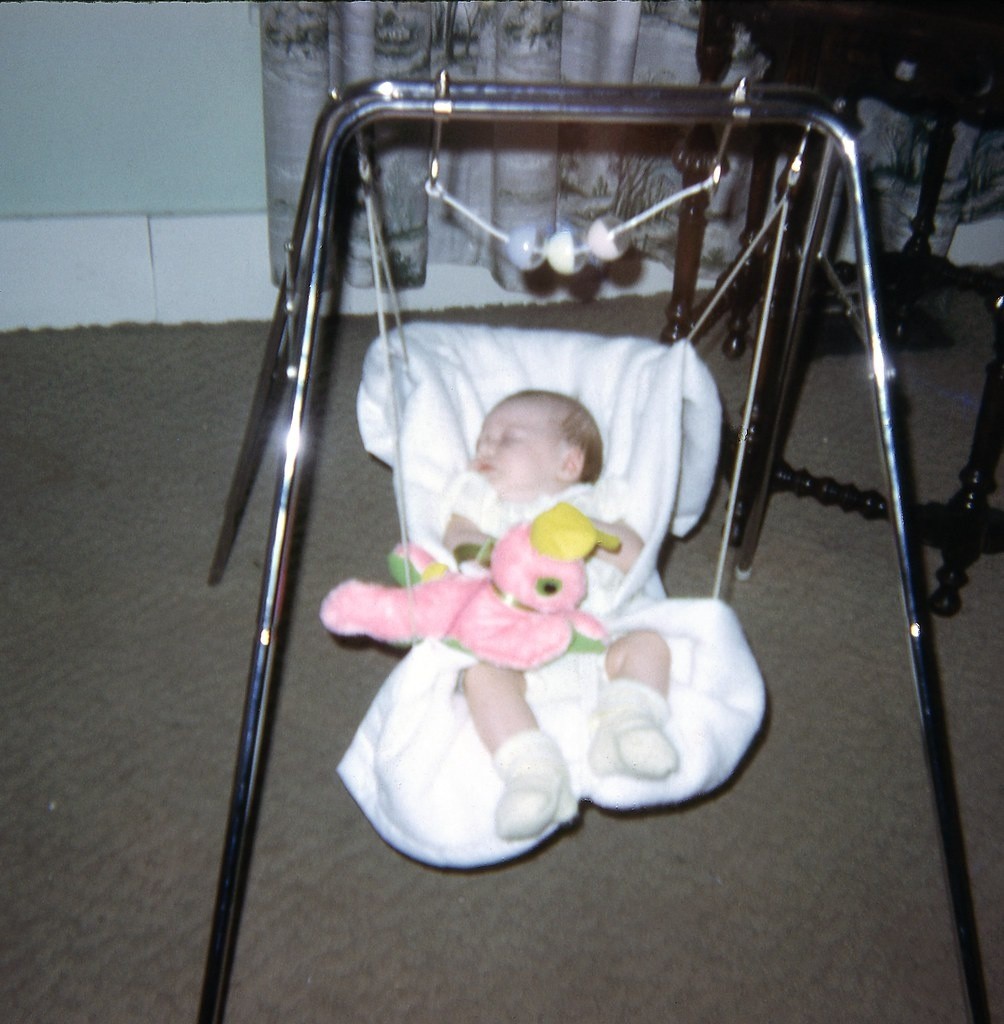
[319,502,620,671]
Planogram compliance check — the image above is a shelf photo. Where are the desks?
[658,0,1003,617]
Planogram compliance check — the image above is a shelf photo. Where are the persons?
[440,391,678,839]
